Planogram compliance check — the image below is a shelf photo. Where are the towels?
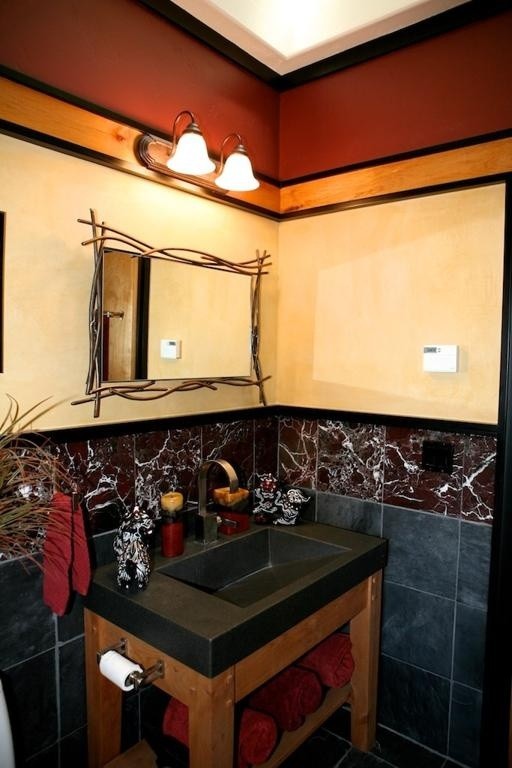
[42,489,90,616]
[162,629,355,768]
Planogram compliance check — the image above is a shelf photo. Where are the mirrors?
[68,206,274,416]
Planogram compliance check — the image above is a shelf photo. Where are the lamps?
[137,110,260,203]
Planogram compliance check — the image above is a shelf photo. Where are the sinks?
[155,527,351,608]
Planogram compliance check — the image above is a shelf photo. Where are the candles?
[161,492,184,512]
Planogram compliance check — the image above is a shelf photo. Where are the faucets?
[194,459,238,542]
